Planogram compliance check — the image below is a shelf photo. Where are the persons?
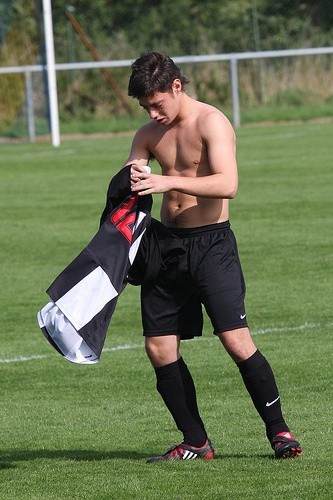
[118,49,303,465]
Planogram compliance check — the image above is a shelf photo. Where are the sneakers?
[145,438,216,463]
[271,431,302,459]
